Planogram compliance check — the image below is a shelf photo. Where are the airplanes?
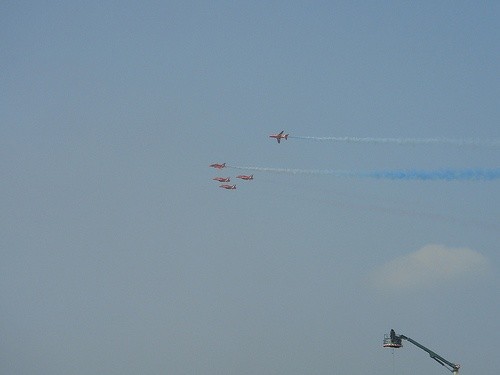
[236,175,253,180]
[209,163,226,169]
[269,131,289,144]
[218,185,237,190]
[213,176,231,182]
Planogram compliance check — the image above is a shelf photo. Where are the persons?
[389,329,396,343]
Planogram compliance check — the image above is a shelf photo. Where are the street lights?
[383,328,460,375]
